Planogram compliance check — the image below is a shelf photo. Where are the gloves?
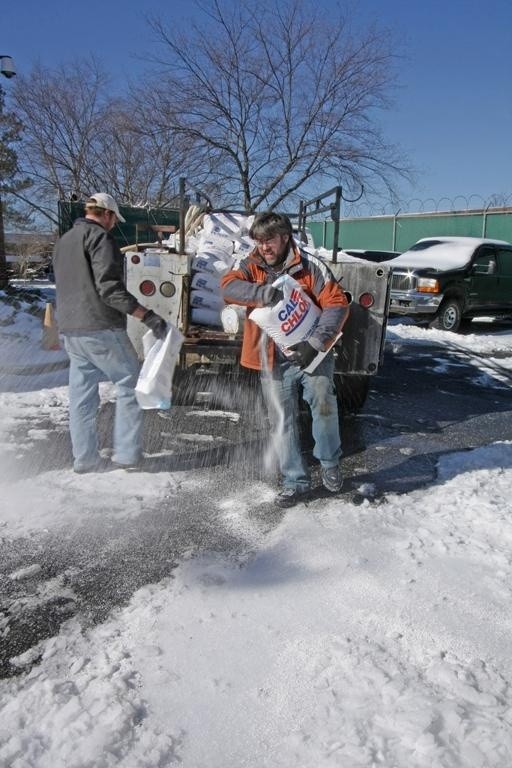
[141,308,168,341]
[287,340,318,371]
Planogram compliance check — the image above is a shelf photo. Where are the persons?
[50,191,168,475]
[219,209,352,510]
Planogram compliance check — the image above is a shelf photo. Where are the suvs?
[341,237,512,330]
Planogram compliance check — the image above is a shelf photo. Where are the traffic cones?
[42,302,59,349]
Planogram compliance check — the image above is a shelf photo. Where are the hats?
[84,192,127,224]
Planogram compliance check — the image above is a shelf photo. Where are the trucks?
[123,177,393,407]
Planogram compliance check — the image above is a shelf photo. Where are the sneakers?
[274,482,310,508]
[73,453,164,476]
[321,468,344,492]
